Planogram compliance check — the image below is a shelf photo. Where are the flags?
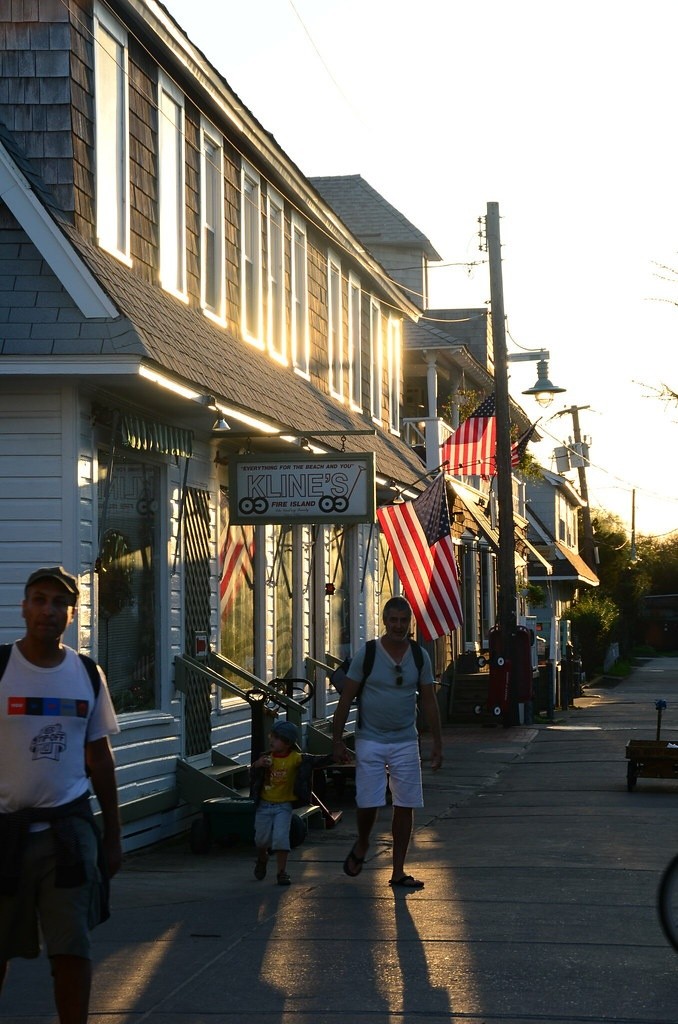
[509,421,539,470]
[220,497,256,633]
[377,470,467,644]
[439,387,500,479]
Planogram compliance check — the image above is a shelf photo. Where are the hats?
[269,718,301,752]
[25,566,79,595]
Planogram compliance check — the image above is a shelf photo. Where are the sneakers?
[276,873,290,884]
[254,855,269,879]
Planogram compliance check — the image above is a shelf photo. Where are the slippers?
[342,841,369,876]
[388,876,425,888]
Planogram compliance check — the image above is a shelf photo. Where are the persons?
[333,594,447,888]
[0,560,125,1024]
[249,718,349,888]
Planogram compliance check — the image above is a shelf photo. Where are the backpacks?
[329,639,423,730]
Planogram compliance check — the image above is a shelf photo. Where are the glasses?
[395,665,403,686]
